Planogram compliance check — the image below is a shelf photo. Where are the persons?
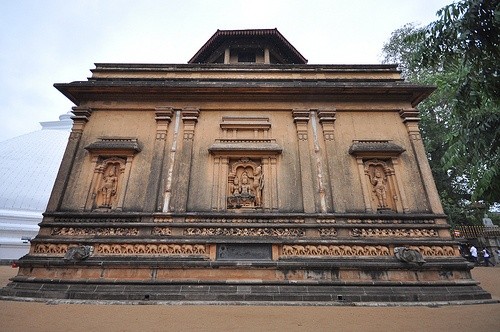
[468,244,482,267]
[367,169,388,208]
[495,247,500,266]
[479,246,496,267]
[232,175,256,197]
[98,166,118,207]
